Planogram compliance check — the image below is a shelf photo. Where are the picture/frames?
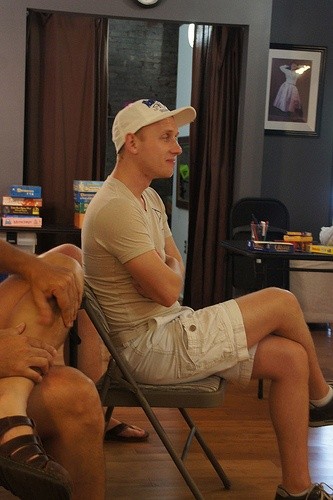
[264,43,327,137]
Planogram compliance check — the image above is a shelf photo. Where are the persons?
[273,62,310,116]
[0,237,105,500]
[81,99,333,500]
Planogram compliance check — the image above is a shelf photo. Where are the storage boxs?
[74,180,104,229]
[304,244,333,256]
[1,185,42,227]
[247,240,294,254]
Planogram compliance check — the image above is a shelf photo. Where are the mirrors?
[176,137,188,210]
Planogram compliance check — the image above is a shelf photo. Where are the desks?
[220,239,333,398]
[0,222,81,244]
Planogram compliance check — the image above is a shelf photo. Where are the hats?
[112,99,197,154]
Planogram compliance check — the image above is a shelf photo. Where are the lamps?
[136,0,162,9]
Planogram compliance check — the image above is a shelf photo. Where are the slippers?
[104,413,149,442]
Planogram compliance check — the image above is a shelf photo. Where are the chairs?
[81,280,232,500]
[230,197,290,293]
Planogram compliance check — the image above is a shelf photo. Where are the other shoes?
[274,481,333,500]
[308,389,333,427]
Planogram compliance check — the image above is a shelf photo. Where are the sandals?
[0,415,72,500]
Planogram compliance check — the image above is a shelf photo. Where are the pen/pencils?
[250,214,269,241]
